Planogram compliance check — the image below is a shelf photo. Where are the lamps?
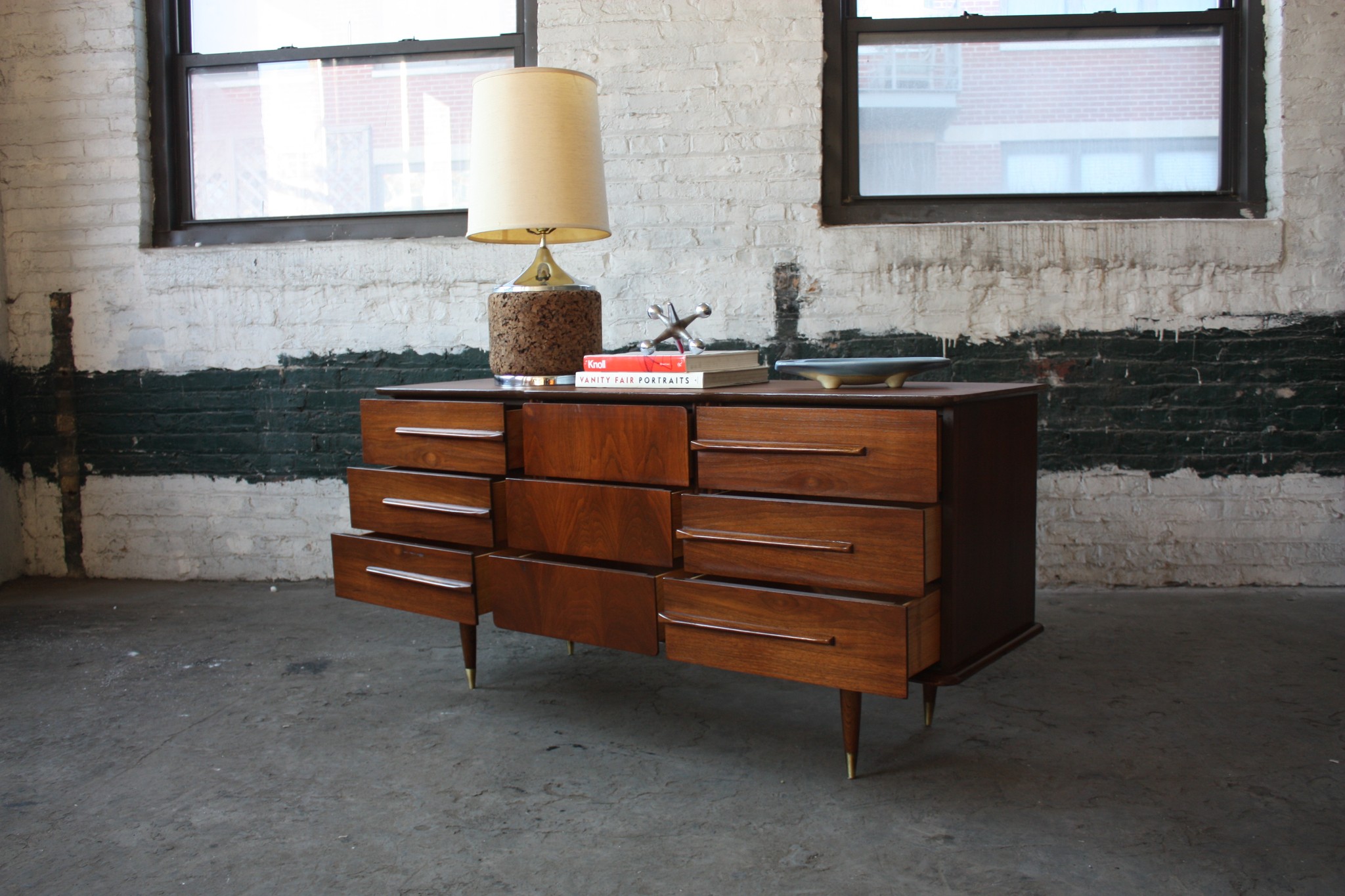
[465,66,612,374]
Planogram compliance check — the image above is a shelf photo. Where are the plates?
[773,356,952,388]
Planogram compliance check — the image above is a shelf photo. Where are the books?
[575,365,770,389]
[583,350,759,372]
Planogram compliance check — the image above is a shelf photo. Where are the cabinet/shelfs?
[330,368,1048,778]
